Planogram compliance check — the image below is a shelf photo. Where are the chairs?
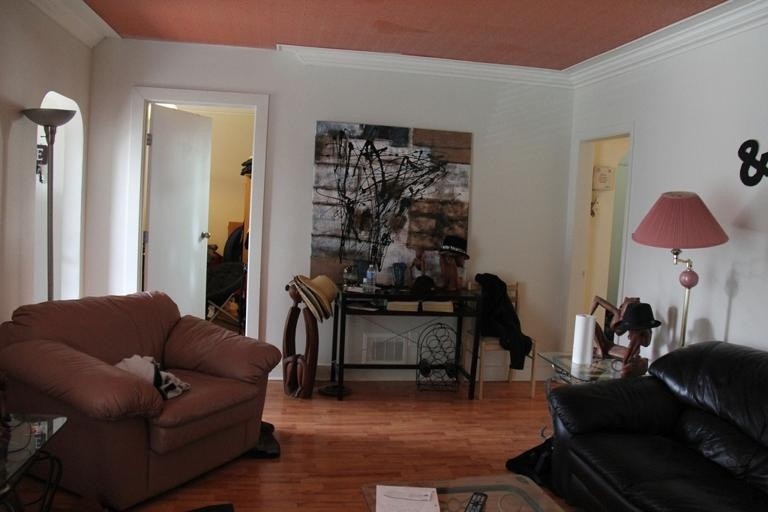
[465,278,537,401]
[0,289,284,509]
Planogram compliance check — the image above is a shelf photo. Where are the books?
[376,485,440,512]
[347,299,454,312]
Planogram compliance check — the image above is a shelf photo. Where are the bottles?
[366,263,377,294]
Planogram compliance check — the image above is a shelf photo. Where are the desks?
[326,281,475,401]
[535,347,627,440]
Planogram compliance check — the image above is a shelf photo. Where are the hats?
[614,302,662,334]
[439,234,470,260]
[293,275,339,322]
[413,274,437,295]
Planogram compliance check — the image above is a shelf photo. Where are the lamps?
[631,190,731,346]
[18,105,75,301]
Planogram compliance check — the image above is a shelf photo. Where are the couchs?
[506,340,768,511]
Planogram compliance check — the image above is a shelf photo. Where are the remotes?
[464,492,488,512]
